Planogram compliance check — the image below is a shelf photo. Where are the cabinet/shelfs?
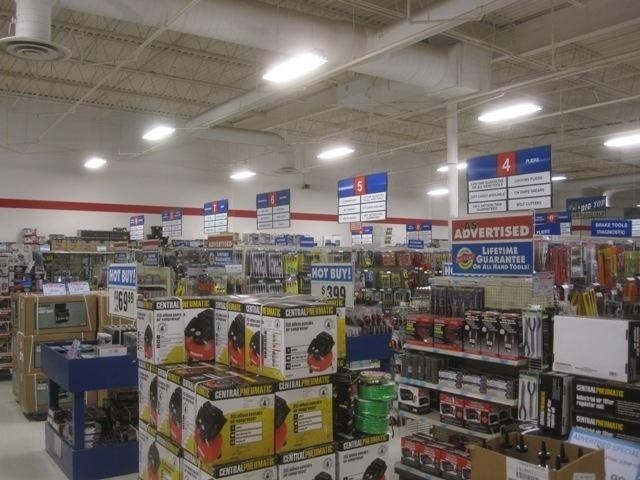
[41,341,139,480]
[394,340,529,479]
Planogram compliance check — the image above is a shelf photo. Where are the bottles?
[555,443,570,470]
[501,431,512,452]
[576,446,583,458]
[482,438,487,449]
[537,441,550,468]
[515,434,529,453]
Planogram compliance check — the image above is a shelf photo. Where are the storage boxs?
[470,431,605,480]
[573,376,639,446]
[566,426,640,480]
[10,291,136,417]
[538,369,574,441]
